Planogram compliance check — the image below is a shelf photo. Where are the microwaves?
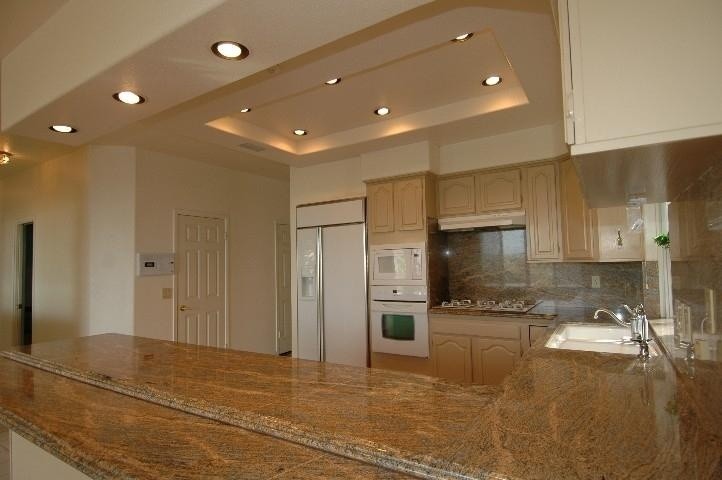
[373,248,424,281]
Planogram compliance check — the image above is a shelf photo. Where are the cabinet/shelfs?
[559,153,602,265]
[522,313,556,355]
[435,169,478,219]
[477,163,526,216]
[595,204,646,264]
[427,307,473,386]
[362,169,437,246]
[551,0,722,207]
[520,158,560,265]
[470,310,522,385]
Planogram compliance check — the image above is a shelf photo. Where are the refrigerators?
[295,198,371,370]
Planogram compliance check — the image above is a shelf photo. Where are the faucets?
[593,303,648,342]
[694,315,711,362]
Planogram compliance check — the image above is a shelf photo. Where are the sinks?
[544,321,662,352]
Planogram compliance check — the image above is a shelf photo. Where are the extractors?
[436,211,525,235]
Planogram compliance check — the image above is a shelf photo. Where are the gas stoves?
[432,298,544,313]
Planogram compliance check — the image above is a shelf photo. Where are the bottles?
[639,339,649,357]
[695,339,710,361]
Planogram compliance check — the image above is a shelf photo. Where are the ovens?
[368,285,429,360]
